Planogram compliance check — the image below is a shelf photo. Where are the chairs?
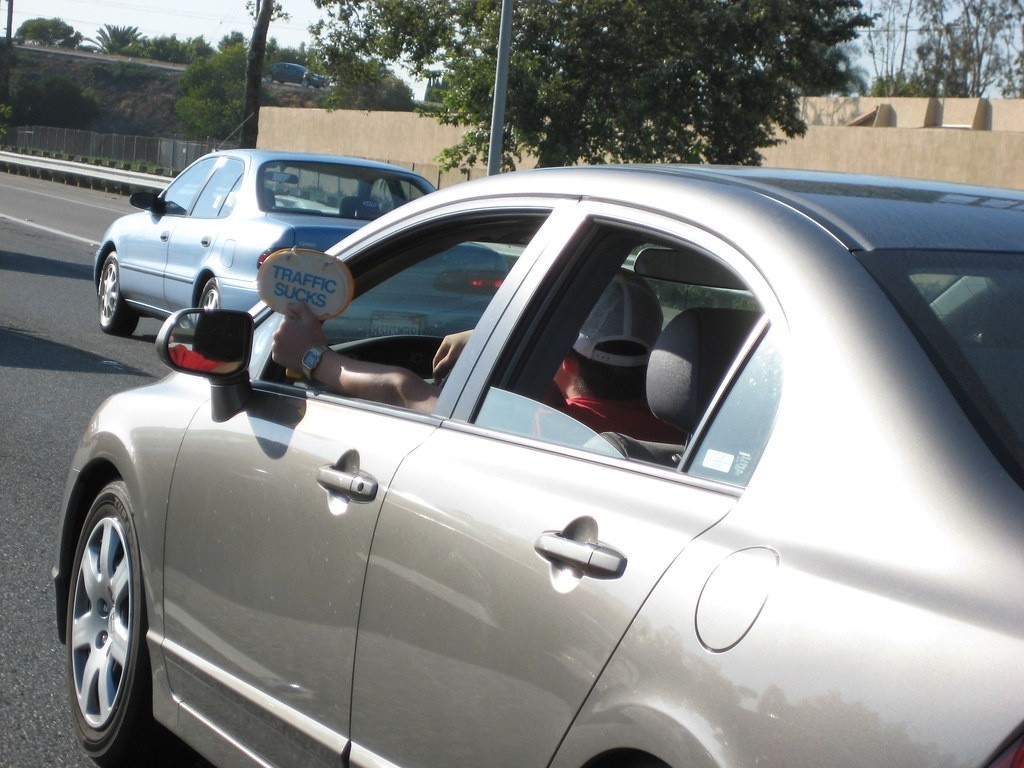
[585,307,782,488]
[217,187,277,215]
[339,195,380,218]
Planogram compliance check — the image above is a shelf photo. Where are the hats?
[570,265,664,368]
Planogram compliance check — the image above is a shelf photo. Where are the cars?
[262,63,330,88]
[93,149,437,336]
[56,164,1024,768]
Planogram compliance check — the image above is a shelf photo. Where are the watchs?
[301,344,333,382]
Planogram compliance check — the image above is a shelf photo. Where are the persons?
[271,267,686,445]
